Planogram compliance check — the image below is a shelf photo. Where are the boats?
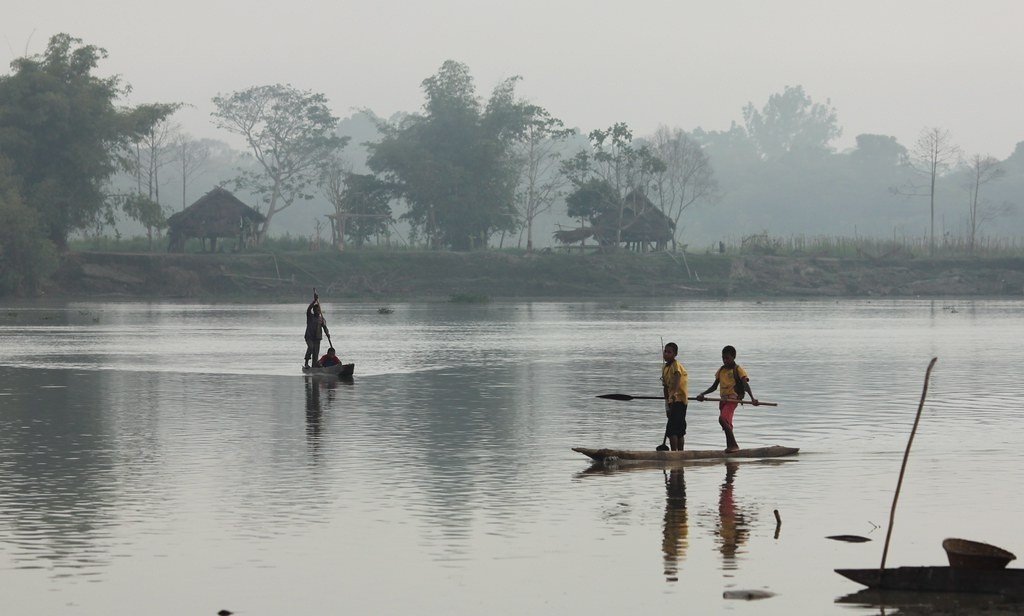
[835,565,1024,588]
[835,588,1024,609]
[301,361,355,377]
[570,444,800,461]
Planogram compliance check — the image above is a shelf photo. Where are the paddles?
[313,288,335,355]
[595,393,778,407]
[655,399,675,451]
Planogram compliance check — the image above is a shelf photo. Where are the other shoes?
[725,446,739,453]
[305,364,310,368]
[312,363,319,367]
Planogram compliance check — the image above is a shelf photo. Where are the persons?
[696,345,759,453]
[304,295,330,369]
[662,342,689,451]
[318,347,342,367]
